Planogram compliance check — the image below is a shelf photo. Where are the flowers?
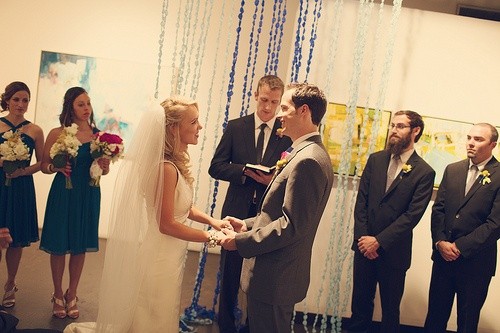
[400,163,412,178]
[275,127,283,137]
[478,170,491,185]
[275,152,289,172]
[0,130,31,186]
[49,123,82,190]
[89,131,126,185]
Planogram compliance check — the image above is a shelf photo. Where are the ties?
[256,124,266,165]
[465,166,478,193]
[286,147,293,152]
[385,154,401,192]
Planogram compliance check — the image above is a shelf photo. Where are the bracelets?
[47,164,55,174]
[206,230,218,248]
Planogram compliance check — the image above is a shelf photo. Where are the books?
[242,163,277,177]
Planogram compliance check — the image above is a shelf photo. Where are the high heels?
[65,289,80,319]
[2,282,16,307]
[52,292,66,318]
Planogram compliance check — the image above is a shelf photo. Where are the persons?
[66,95,235,333]
[350,111,436,333]
[39,87,111,319]
[0,81,44,308]
[219,82,334,333]
[424,122,500,333]
[208,75,293,333]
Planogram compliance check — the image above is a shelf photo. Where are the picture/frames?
[318,102,393,176]
[414,115,475,190]
